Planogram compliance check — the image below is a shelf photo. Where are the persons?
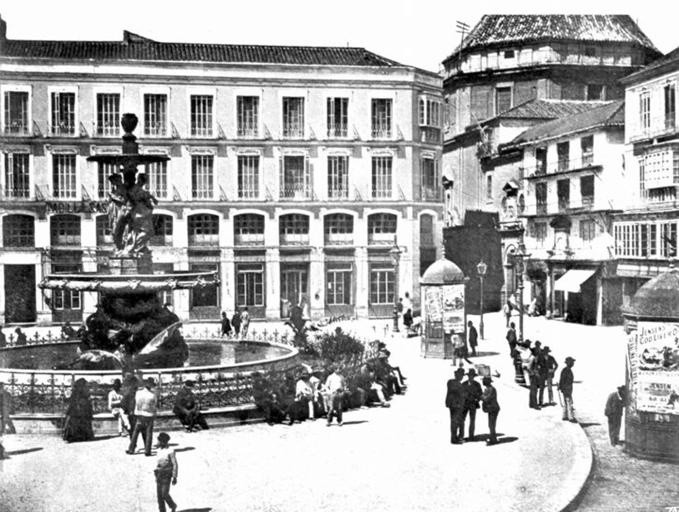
[59,374,210,456]
[604,385,625,446]
[131,174,158,253]
[251,339,407,427]
[107,173,132,251]
[219,306,252,339]
[152,431,178,511]
[444,317,581,444]
[396,293,423,334]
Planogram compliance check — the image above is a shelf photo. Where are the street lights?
[476,258,489,328]
[508,233,532,341]
[389,235,401,309]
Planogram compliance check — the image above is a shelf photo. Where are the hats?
[143,377,156,387]
[454,367,465,376]
[524,339,551,352]
[158,432,170,442]
[466,367,479,376]
[565,356,576,363]
[617,385,625,396]
[481,375,494,383]
